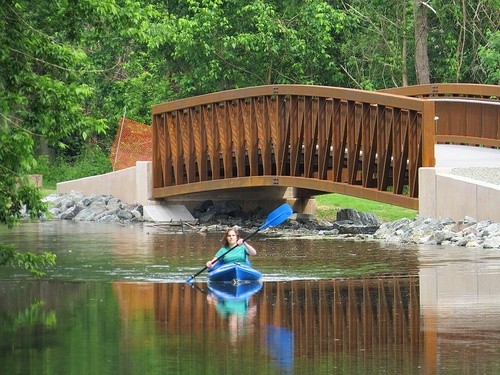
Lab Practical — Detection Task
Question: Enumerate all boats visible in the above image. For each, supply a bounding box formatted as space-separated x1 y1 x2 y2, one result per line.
207 263 262 280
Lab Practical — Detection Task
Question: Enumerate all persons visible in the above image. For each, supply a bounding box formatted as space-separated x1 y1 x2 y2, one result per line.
206 228 257 268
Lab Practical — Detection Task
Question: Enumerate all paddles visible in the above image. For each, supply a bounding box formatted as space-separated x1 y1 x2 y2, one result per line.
186 203 293 283
